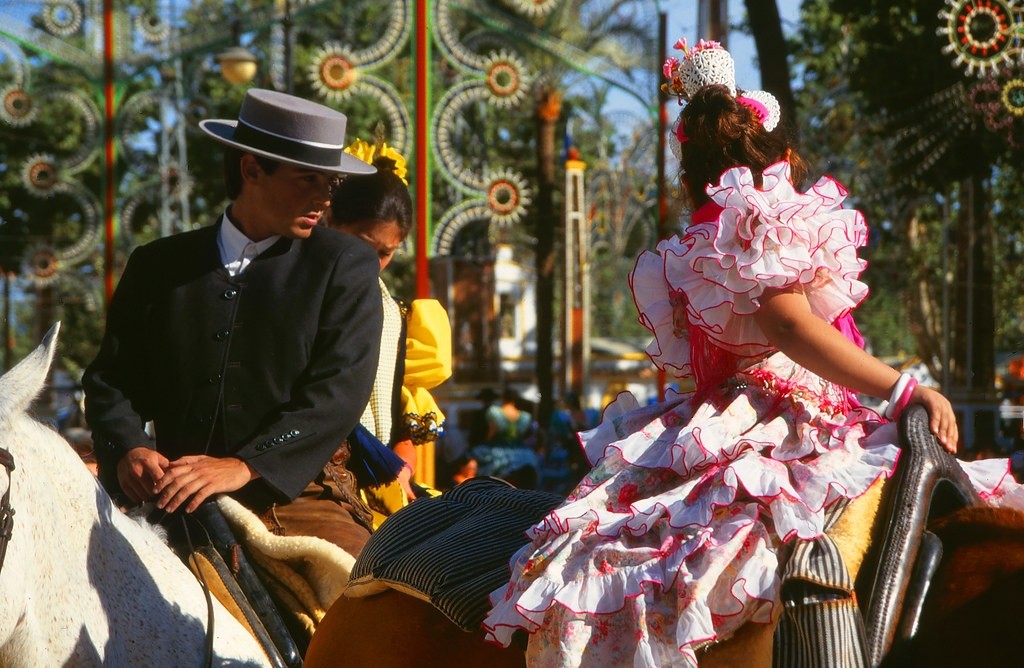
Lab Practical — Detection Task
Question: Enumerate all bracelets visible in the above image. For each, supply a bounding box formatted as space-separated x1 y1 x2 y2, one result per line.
886 373 918 423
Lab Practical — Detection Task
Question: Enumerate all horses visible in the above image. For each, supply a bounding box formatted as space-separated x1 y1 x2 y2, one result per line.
300 459 1024 668
0 322 393 667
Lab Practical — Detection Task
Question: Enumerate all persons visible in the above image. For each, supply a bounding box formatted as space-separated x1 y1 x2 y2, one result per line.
459 388 601 488
80 87 383 616
482 32 959 668
321 136 417 535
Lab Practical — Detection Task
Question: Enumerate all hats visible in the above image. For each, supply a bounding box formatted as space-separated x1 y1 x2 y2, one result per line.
662 35 782 161
198 87 378 178
